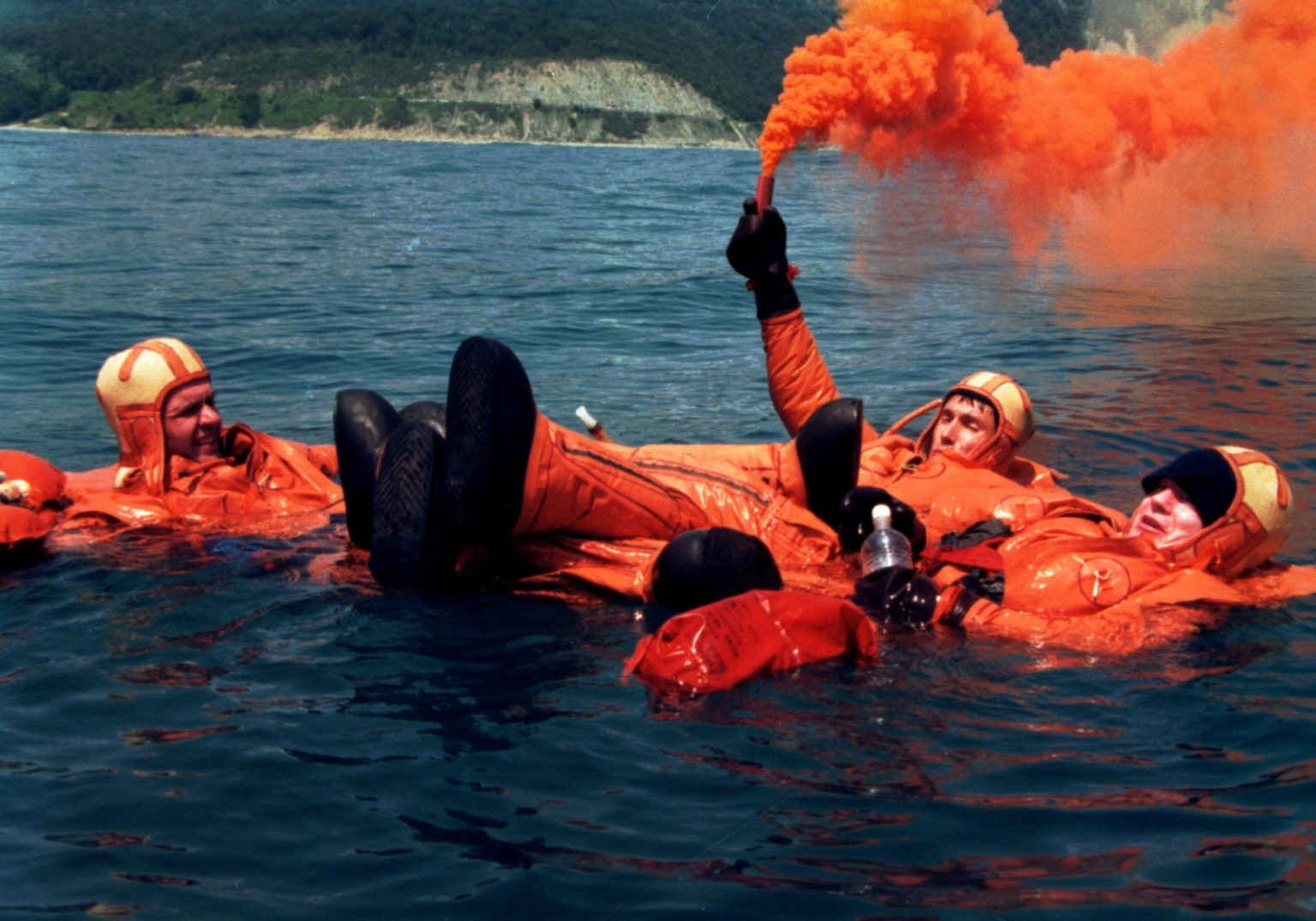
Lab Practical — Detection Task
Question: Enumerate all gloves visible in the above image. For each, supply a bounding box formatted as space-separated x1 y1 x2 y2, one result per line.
838 486 926 561
727 197 802 318
848 565 938 630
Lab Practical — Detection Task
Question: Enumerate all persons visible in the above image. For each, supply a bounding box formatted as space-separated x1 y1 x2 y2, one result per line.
46 196 1295 675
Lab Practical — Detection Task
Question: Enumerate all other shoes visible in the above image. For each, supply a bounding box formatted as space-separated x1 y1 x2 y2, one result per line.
436 338 536 550
797 398 865 535
333 389 403 545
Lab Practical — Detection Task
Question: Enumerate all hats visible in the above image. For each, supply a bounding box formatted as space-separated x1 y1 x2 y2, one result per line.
1142 448 1237 528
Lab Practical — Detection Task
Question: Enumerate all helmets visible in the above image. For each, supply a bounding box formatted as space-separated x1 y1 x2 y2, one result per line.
1167 445 1296 580
915 371 1037 474
96 336 210 497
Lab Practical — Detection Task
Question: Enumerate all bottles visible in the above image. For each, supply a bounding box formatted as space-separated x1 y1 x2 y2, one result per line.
859 502 914 576
